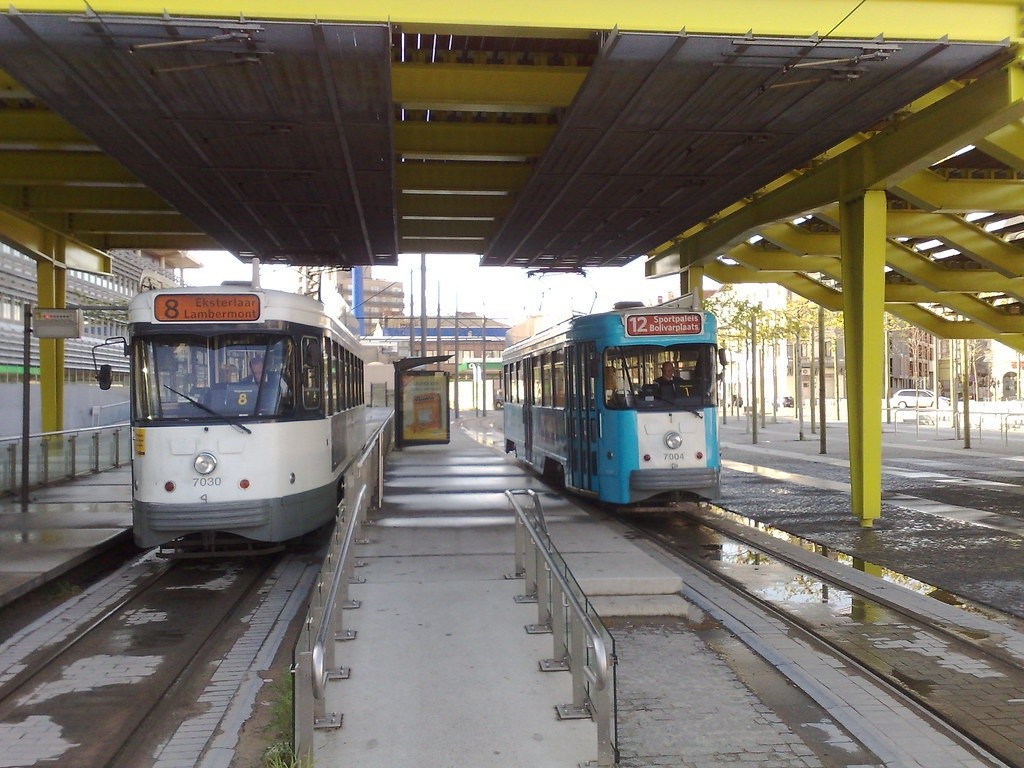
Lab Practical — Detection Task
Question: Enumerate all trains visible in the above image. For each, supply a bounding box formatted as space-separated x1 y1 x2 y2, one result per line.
125 285 369 561
501 300 728 510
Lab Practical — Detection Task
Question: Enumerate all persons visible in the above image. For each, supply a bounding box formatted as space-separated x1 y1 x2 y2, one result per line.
243 357 288 399
653 363 687 396
938 380 945 398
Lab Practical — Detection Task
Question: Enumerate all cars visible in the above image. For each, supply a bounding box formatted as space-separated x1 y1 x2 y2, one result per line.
771 396 794 408
891 388 945 409
